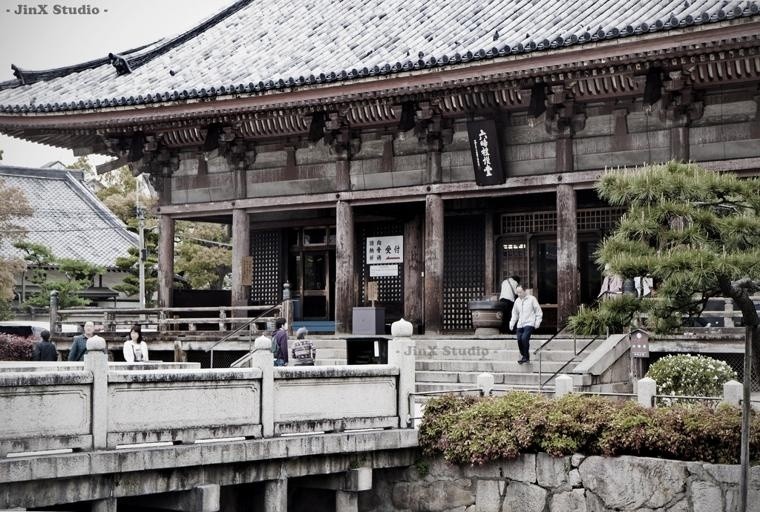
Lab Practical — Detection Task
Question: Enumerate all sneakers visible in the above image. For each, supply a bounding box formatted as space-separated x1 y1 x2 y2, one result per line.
518 358 529 362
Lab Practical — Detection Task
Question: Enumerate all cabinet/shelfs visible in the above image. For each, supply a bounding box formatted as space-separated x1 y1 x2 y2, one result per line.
352 306 385 335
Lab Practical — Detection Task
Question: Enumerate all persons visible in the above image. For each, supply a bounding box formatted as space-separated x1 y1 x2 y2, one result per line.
290 327 317 367
499 275 519 335
68 321 96 361
596 262 713 338
122 325 149 363
31 329 58 363
509 284 544 365
269 318 289 367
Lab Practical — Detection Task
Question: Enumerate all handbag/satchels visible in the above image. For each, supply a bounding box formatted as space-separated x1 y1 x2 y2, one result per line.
271 337 280 353
